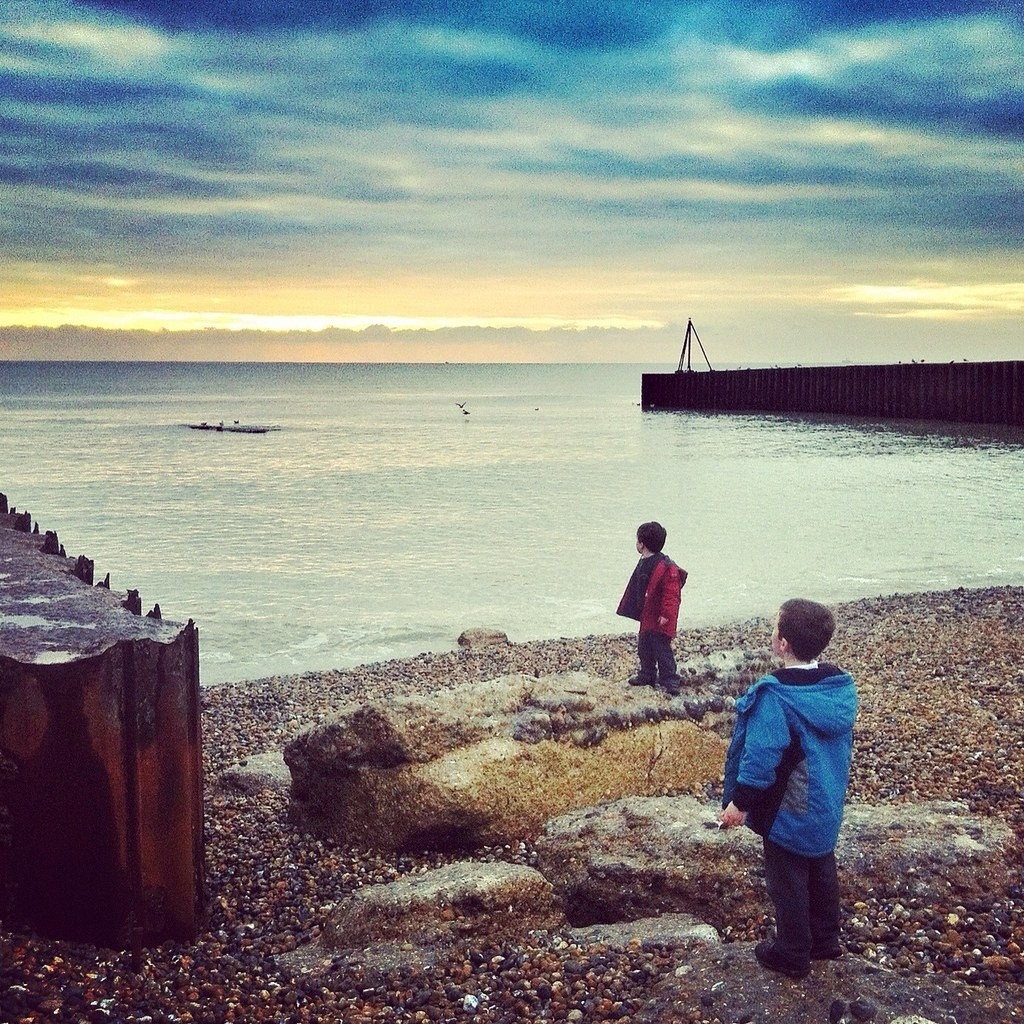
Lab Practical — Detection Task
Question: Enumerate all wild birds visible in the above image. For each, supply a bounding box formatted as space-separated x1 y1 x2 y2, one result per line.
455 402 467 408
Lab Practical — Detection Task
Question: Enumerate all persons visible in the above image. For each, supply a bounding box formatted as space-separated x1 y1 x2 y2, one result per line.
719 598 859 977
614 521 682 694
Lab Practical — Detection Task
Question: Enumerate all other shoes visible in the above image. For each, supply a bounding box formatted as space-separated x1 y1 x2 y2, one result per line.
810 945 843 959
755 942 811 978
665 682 680 696
628 675 656 688
